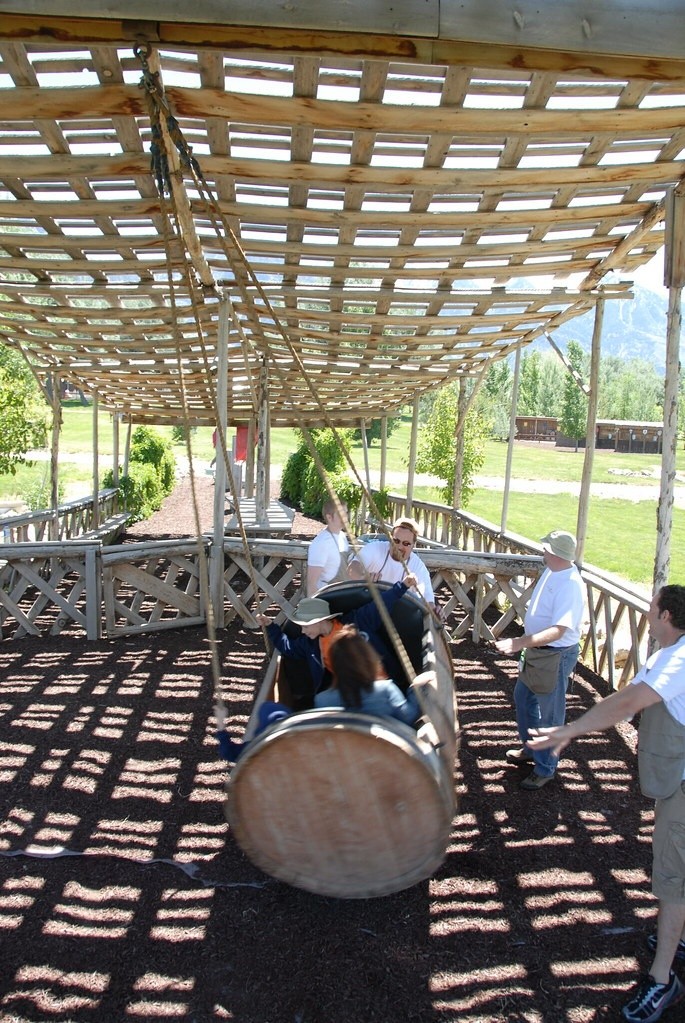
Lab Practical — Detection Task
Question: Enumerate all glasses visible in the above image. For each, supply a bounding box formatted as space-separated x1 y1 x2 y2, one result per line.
391 537 413 546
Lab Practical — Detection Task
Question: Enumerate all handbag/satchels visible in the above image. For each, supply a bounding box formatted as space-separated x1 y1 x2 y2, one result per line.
515 646 561 694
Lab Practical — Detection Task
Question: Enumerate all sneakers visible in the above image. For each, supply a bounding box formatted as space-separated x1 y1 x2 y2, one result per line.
505 748 534 762
618 968 684 1023
647 934 685 958
520 771 554 790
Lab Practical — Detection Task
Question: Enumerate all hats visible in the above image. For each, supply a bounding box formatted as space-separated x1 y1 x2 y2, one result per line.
392 518 420 538
283 598 343 626
540 529 577 561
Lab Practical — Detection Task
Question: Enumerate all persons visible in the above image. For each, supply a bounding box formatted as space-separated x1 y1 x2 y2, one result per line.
346 522 447 621
307 500 350 598
526 586 685 1023
495 530 586 789
255 573 434 724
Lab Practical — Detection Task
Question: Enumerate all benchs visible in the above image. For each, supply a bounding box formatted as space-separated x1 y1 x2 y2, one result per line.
67 512 132 546
